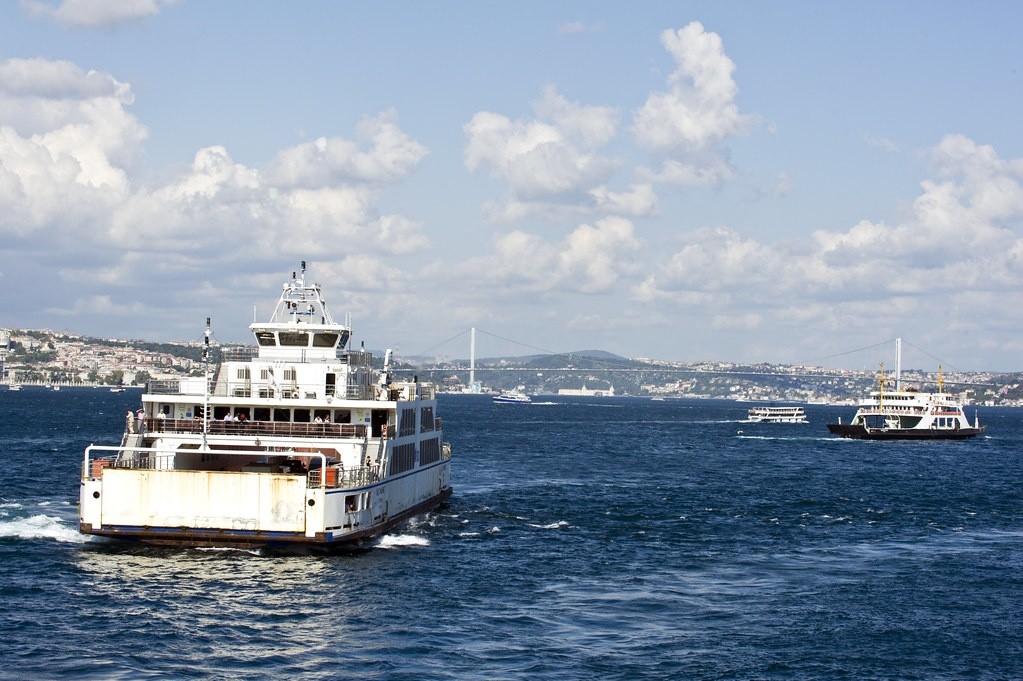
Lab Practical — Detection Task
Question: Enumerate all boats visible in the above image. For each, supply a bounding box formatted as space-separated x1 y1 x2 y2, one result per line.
77 258 455 546
747 407 811 424
826 361 988 440
492 386 533 405
